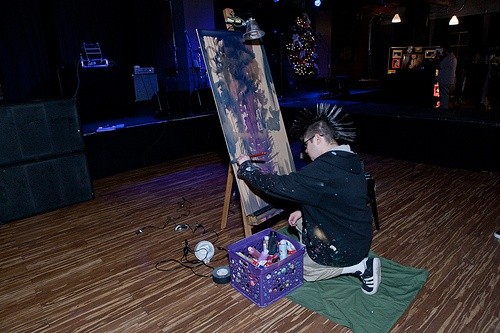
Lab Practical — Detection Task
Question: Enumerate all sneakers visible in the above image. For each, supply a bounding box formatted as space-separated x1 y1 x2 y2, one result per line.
359 257 381 295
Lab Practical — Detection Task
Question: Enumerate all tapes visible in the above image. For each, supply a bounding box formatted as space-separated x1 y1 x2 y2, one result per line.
213 265 230 284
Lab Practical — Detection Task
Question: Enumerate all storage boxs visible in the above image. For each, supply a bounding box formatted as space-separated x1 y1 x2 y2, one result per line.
228 228 304 307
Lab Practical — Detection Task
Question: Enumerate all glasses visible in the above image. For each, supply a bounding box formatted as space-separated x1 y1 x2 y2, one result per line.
303 134 324 148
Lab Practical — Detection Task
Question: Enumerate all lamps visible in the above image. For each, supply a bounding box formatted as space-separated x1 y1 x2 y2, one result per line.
227 10 264 40
391 14 402 23
448 0 459 26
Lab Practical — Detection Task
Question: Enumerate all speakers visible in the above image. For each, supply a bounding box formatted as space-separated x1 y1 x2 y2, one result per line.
0 99 95 225
263 40 289 97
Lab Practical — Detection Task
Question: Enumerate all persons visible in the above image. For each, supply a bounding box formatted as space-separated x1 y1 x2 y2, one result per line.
436 44 457 111
236 102 381 295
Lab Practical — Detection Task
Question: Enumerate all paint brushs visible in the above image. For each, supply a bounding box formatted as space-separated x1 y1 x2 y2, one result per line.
229 152 267 165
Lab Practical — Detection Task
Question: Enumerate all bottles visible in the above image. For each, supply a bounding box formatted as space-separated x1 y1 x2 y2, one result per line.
268 231 278 263
279 240 288 261
263 235 270 252
247 246 260 260
